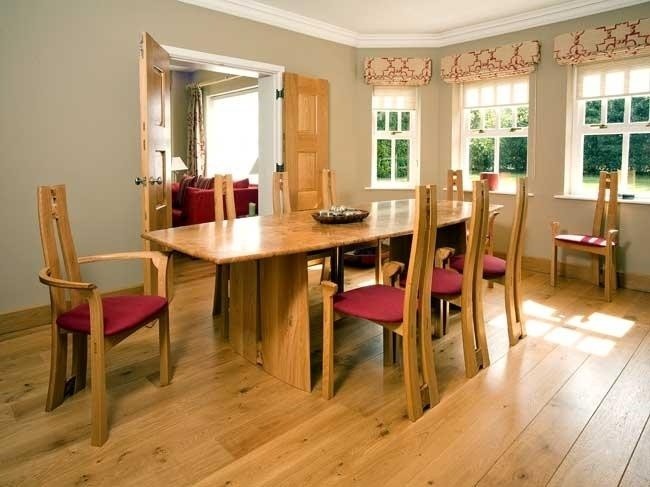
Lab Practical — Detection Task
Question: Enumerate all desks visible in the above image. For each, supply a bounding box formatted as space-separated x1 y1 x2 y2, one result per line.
142 199 503 392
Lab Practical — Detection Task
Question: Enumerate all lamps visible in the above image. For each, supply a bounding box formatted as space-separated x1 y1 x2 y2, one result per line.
250 158 258 174
172 156 188 182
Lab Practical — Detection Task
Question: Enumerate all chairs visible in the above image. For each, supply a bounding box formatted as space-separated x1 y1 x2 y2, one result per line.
321 186 439 421
318 169 337 208
39 183 173 446
392 178 490 377
444 169 465 201
271 171 292 214
446 174 531 346
550 171 619 302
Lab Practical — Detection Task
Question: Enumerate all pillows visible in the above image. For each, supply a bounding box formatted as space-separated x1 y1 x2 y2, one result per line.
177 174 248 208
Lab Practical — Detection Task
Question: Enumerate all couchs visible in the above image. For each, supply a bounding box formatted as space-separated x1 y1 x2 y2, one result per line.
172 183 258 225
